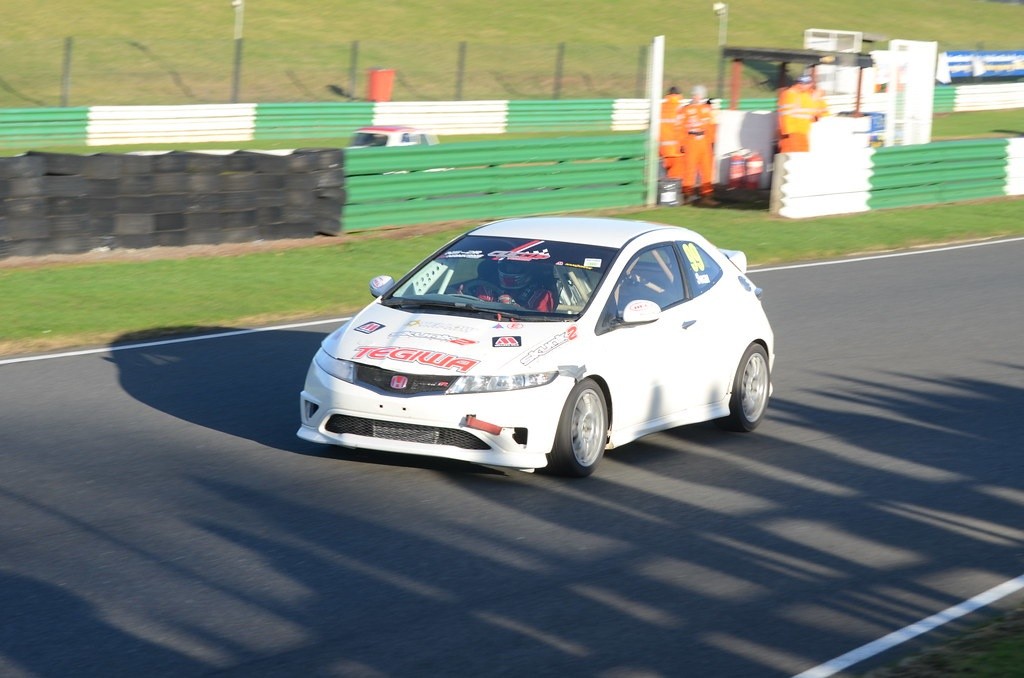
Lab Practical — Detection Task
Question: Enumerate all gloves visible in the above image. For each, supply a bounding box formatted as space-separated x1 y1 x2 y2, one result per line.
498 294 519 308
457 284 465 295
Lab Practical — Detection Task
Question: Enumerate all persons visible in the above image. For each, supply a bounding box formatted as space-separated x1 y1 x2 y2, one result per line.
776 74 827 152
457 259 554 312
659 85 721 206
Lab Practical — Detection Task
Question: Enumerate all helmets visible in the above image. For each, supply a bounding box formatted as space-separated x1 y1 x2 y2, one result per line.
498 259 530 290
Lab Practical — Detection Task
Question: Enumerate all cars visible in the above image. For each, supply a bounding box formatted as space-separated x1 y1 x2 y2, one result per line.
296 216 776 479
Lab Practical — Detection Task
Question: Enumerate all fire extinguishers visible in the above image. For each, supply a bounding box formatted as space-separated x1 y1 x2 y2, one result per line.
724 148 763 190
